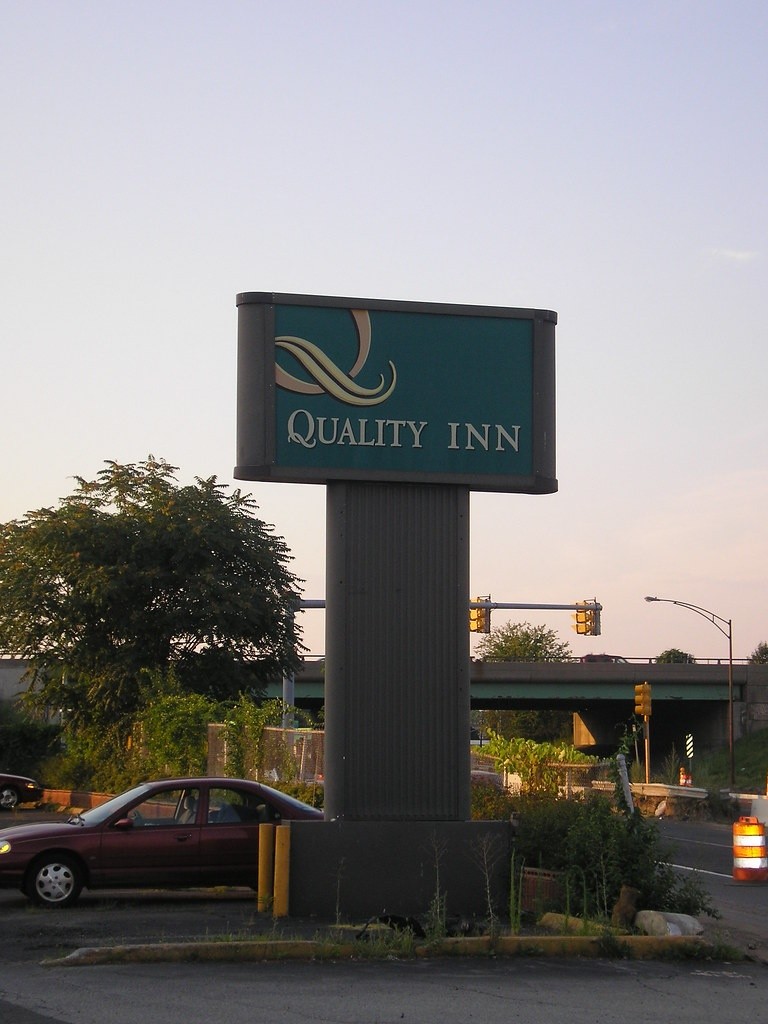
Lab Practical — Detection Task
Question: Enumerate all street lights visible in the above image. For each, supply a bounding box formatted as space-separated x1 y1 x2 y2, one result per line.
644 596 733 792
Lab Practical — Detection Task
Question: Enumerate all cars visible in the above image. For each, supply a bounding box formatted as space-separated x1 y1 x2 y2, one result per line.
0 774 43 811
0 776 324 910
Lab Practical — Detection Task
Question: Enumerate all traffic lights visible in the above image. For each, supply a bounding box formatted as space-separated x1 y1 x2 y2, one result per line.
571 602 595 636
635 684 651 716
469 599 486 633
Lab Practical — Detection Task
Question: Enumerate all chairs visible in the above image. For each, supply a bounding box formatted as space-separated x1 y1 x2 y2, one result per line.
177 795 195 824
186 798 199 824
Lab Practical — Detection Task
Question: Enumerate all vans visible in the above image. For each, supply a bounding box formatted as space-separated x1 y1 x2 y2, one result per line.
582 654 629 663
